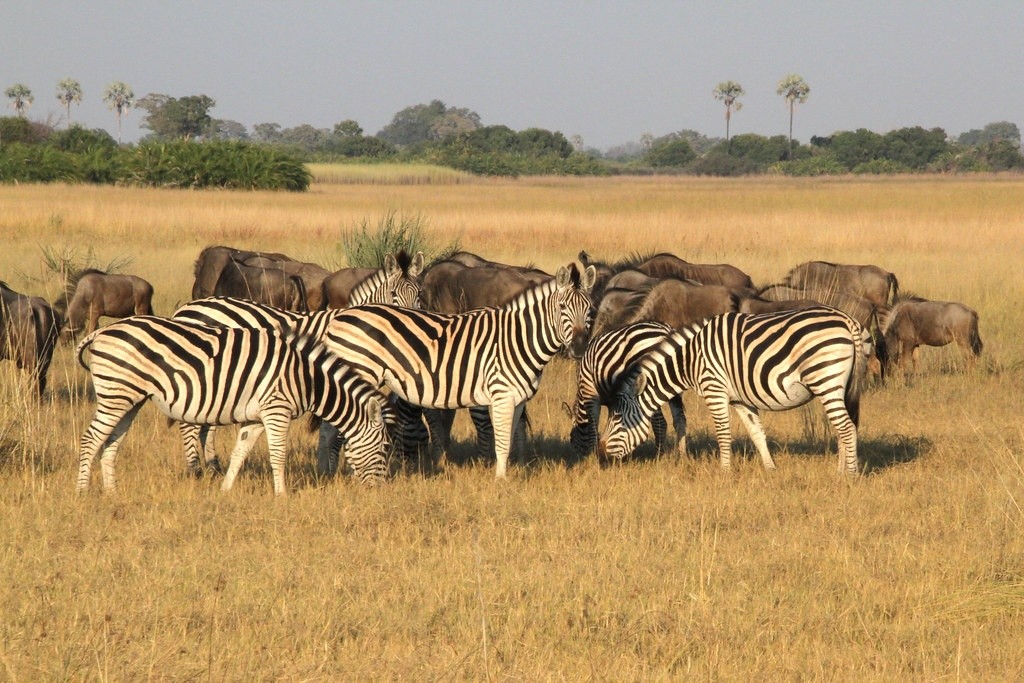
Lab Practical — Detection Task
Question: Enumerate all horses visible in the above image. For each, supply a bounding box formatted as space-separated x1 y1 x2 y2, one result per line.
1 244 983 399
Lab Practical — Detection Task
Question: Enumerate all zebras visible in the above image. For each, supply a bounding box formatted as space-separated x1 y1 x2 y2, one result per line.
73 250 867 493
